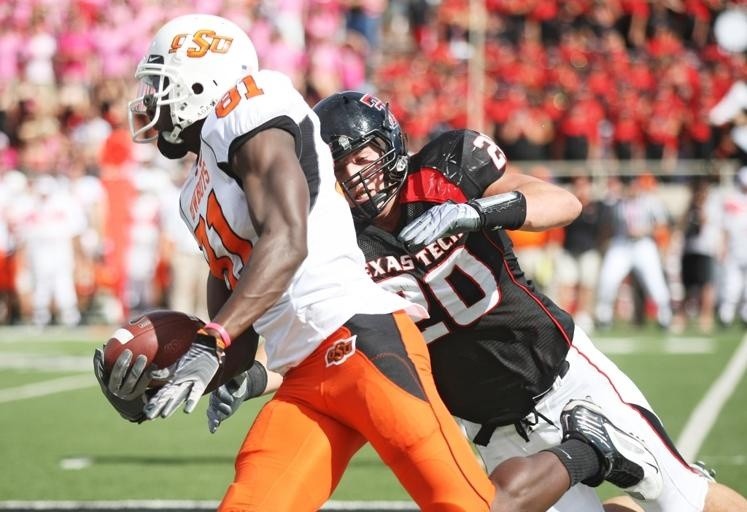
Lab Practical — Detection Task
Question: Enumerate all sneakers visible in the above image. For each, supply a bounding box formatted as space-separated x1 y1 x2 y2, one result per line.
559 399 664 503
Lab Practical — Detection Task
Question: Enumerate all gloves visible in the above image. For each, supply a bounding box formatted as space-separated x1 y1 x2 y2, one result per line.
398 202 483 247
142 342 221 419
93 347 155 423
207 358 269 434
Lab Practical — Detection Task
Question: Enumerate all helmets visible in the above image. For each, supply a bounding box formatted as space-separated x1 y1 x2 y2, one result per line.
312 91 407 226
127 12 259 160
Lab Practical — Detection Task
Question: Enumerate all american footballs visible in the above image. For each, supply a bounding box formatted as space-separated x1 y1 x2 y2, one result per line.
103 310 206 388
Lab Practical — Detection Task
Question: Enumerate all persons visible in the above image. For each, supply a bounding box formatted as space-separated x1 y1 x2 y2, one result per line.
206 90 747 512
1 3 747 331
95 14 664 512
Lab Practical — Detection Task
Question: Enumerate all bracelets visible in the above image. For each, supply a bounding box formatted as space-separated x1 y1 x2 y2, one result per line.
204 322 233 347
